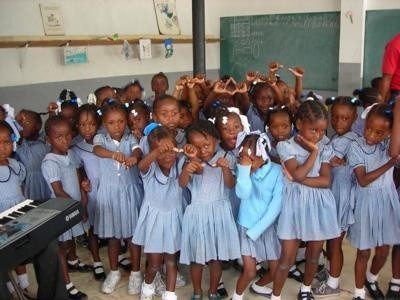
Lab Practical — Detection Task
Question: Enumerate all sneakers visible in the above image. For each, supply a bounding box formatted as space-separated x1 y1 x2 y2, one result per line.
174 271 186 289
162 289 178 300
101 270 121 294
128 270 144 295
312 281 340 297
141 280 155 300
153 271 166 296
18 282 37 299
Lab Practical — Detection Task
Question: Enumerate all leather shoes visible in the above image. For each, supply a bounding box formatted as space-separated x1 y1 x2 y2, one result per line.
364 276 385 299
92 265 107 281
192 289 203 300
117 258 132 269
254 263 267 278
67 257 94 272
297 288 315 300
232 259 244 274
217 283 228 297
65 284 87 300
249 284 273 298
288 266 305 283
352 293 365 300
386 280 400 300
208 287 221 300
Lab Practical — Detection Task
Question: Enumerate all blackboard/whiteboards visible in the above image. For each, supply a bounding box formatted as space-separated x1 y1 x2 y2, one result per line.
363 9 400 87
220 11 340 91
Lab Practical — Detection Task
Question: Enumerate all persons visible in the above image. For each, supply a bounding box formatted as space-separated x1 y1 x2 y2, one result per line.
144 73 169 113
347 103 399 300
215 107 251 271
375 39 400 103
13 109 52 199
88 85 120 125
128 102 151 141
174 61 304 116
0 120 38 300
311 96 358 297
112 88 130 111
389 94 400 300
271 94 342 300
92 103 142 294
41 116 95 300
139 94 192 294
176 100 192 129
0 106 23 154
265 105 294 166
246 84 275 133
60 100 84 148
370 77 384 93
352 89 384 112
137 126 203 300
71 104 133 280
125 79 145 102
178 118 241 300
229 130 283 300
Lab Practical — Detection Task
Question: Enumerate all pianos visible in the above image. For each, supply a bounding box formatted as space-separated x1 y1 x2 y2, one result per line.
0 196 86 271
